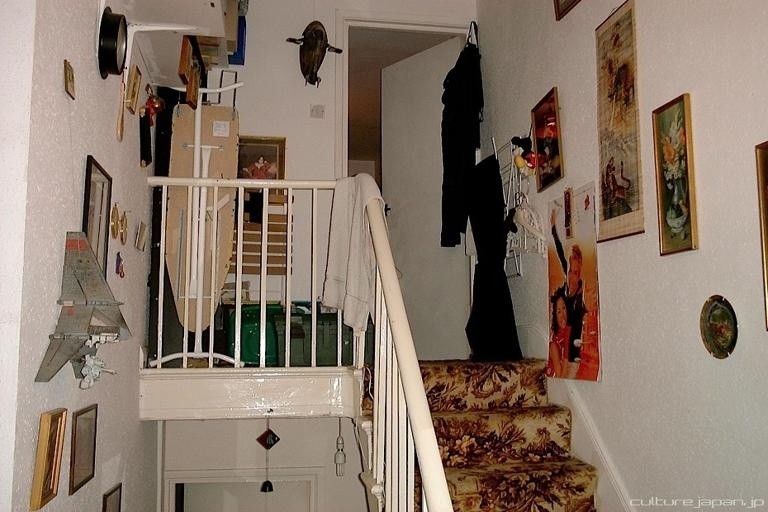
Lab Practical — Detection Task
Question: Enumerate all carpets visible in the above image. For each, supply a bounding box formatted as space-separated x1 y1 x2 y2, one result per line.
361 358 601 512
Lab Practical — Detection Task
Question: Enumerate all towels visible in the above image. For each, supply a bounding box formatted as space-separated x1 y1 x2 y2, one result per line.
320 175 389 337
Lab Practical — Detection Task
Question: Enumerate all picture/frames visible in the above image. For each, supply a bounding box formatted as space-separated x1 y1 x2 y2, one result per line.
31 402 122 511
63 58 75 102
124 64 142 115
530 87 565 193
238 135 286 206
651 92 700 257
82 154 112 282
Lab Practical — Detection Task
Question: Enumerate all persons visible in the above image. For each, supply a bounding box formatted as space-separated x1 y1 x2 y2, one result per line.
549 291 573 379
243 154 278 224
548 206 587 380
539 121 555 175
